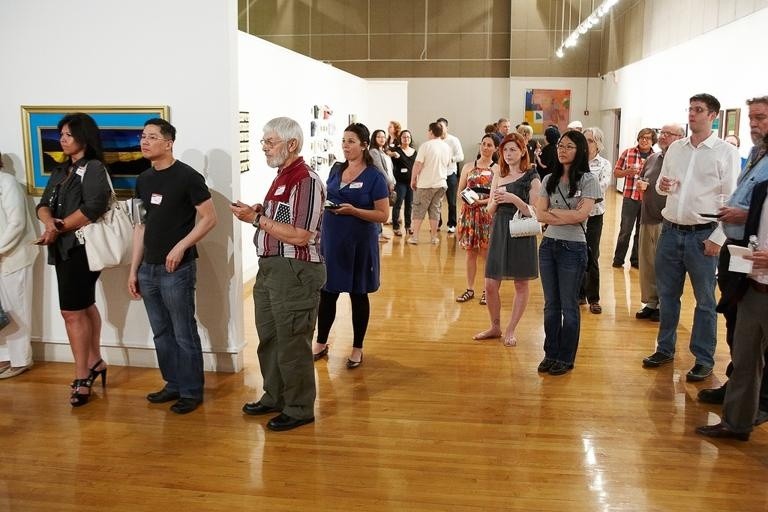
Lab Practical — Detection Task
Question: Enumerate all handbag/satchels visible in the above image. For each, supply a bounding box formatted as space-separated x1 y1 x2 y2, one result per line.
585 232 599 271
509 216 542 239
75 197 133 273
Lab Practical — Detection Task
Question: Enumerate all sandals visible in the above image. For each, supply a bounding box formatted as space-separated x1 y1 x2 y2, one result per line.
456 288 474 302
479 290 486 305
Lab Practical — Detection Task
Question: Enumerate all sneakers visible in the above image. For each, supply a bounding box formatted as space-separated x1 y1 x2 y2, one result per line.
472 330 502 340
643 352 674 367
578 299 587 304
590 302 601 313
613 262 622 267
687 363 713 381
538 358 573 375
636 307 660 322
632 263 639 269
503 336 516 347
375 219 456 244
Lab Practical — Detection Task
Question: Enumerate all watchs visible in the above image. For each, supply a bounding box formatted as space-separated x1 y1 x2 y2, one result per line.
251 213 262 228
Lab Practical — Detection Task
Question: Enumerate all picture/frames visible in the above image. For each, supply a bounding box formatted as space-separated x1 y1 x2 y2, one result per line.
712 110 723 138
725 108 741 137
523 88 572 136
20 105 169 201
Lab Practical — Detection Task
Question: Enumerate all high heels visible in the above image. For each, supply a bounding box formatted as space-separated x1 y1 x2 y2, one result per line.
70 359 106 406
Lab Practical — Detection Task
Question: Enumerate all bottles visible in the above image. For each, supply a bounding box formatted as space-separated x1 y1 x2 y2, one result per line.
748 234 763 252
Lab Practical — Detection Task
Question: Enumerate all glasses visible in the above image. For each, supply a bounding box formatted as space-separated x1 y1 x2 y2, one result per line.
640 135 653 141
660 131 681 138
555 143 577 150
137 133 169 143
260 139 289 149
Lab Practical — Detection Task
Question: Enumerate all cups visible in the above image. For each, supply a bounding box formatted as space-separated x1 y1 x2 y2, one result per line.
497 186 506 191
667 176 679 192
714 194 729 216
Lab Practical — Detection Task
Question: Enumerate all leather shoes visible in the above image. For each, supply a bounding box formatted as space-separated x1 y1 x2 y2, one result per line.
754 409 768 425
266 413 315 430
0 359 33 378
170 398 203 414
243 401 278 415
696 421 749 441
313 345 328 361
697 380 728 404
346 349 363 369
146 387 181 403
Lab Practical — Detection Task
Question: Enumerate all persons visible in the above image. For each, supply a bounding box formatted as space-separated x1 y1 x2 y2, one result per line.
478 120 585 176
129 119 217 413
473 133 541 348
229 117 326 431
613 94 745 382
31 112 114 408
370 117 465 246
696 94 768 440
455 134 501 306
576 127 613 314
0 150 38 379
537 130 603 374
314 122 389 368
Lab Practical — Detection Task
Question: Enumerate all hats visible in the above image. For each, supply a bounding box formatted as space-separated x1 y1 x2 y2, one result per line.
567 120 582 129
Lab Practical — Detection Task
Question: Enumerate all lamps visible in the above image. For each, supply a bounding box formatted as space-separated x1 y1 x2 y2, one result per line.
555 0 619 58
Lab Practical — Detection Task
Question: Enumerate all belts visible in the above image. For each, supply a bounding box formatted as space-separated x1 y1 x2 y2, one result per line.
747 278 768 294
665 219 717 232
